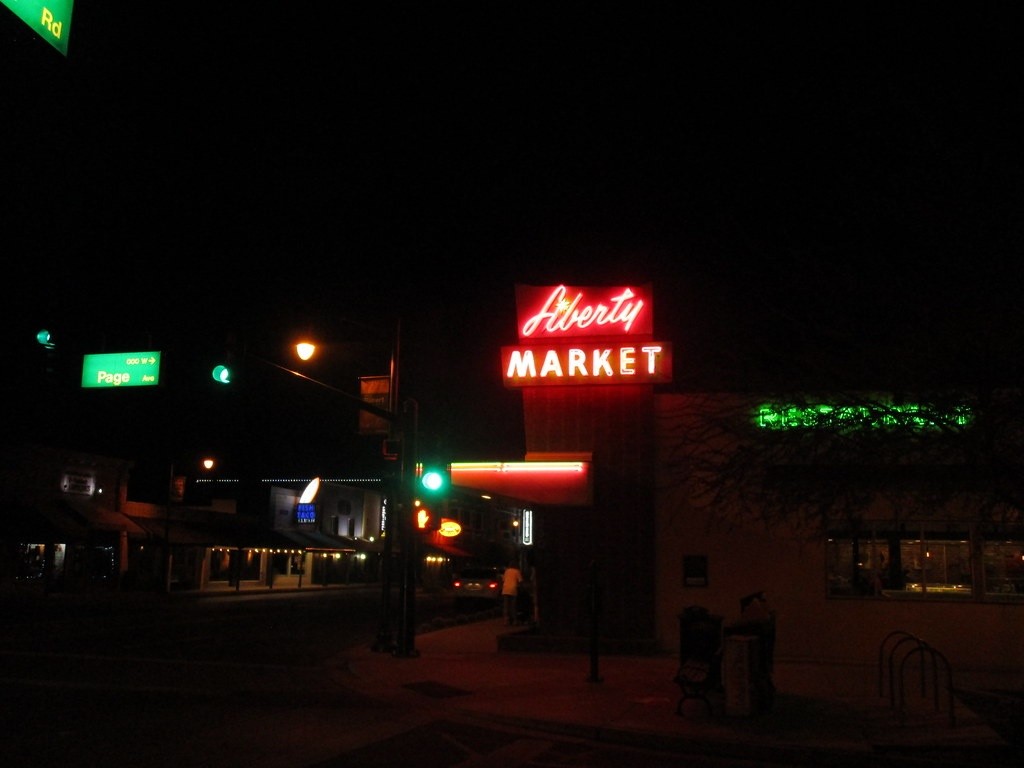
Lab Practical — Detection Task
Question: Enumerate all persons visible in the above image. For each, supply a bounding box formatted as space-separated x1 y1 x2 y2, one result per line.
498 559 532 626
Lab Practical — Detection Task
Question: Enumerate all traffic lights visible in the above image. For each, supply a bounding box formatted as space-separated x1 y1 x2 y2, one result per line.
209 362 233 386
418 467 449 498
35 327 55 349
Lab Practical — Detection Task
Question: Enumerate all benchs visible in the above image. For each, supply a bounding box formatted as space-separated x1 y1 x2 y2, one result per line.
673 644 727 718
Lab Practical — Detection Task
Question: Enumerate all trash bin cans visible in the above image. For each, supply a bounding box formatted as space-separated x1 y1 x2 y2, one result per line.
678 606 720 693
720 613 776 719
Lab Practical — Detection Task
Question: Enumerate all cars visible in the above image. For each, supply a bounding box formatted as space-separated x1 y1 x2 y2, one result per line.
449 567 502 609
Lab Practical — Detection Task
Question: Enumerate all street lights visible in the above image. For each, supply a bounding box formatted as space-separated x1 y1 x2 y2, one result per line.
165 457 215 592
294 338 399 651
